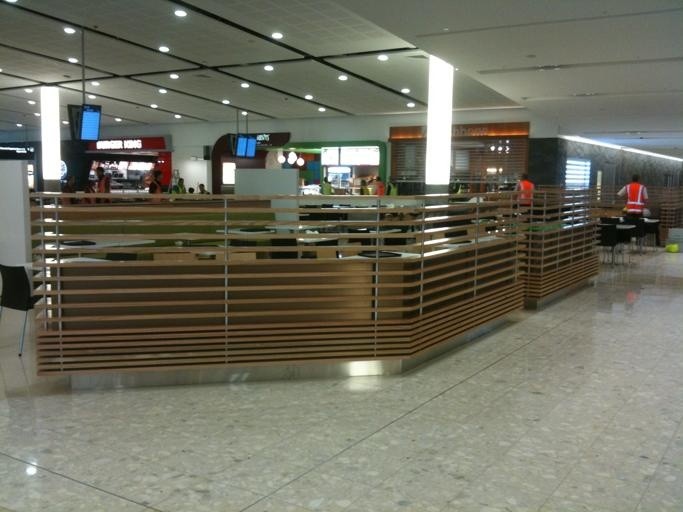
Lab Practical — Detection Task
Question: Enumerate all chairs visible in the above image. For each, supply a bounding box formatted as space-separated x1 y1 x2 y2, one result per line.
0 264 43 356
595 207 661 266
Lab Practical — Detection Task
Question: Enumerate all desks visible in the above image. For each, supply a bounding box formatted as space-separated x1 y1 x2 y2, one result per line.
341 234 517 356
216 225 349 259
31 239 155 257
518 217 593 298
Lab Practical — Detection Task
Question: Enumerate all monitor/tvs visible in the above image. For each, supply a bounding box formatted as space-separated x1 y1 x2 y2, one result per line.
78 103 101 141
67 104 82 140
227 133 257 158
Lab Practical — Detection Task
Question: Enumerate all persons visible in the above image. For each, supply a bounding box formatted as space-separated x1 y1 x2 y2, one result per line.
148 170 211 194
92 168 109 194
320 177 332 194
616 174 649 223
516 173 536 215
360 176 398 196
